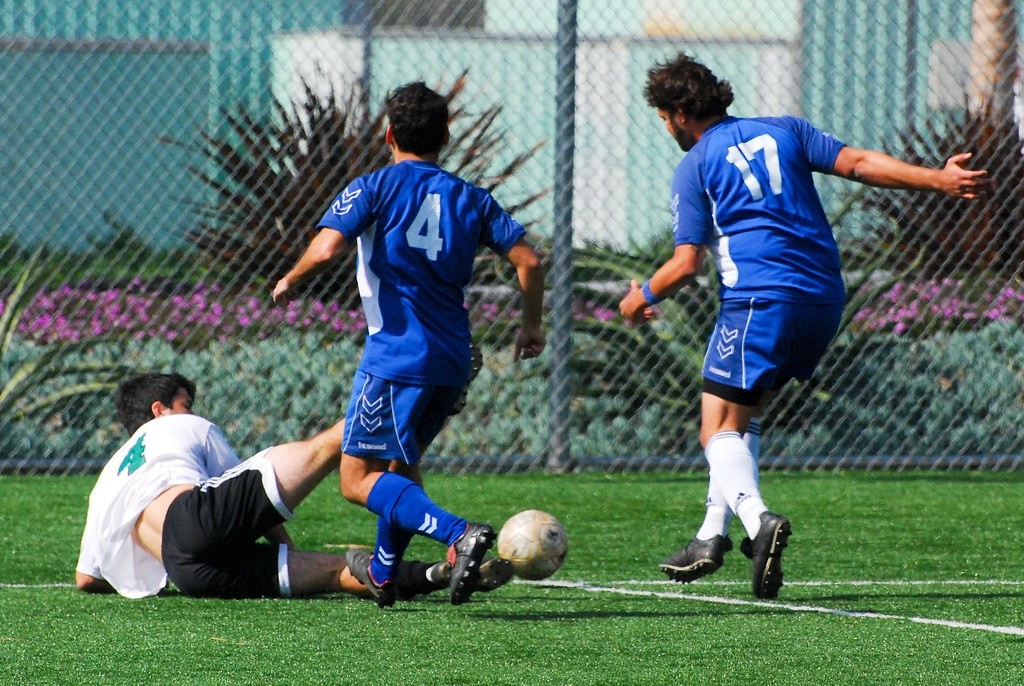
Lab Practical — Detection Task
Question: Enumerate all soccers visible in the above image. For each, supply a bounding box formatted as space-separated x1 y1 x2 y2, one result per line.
496 507 570 581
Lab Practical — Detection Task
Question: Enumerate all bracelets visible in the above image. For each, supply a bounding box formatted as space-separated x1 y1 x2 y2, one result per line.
642 280 658 305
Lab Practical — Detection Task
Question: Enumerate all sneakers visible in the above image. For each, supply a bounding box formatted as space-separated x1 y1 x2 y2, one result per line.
446 343 483 417
658 534 733 585
740 511 792 599
346 550 396 609
431 557 514 592
446 522 498 605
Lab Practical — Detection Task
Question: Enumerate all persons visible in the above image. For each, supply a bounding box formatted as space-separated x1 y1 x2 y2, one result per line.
72 372 514 599
269 80 546 611
618 49 995 600
